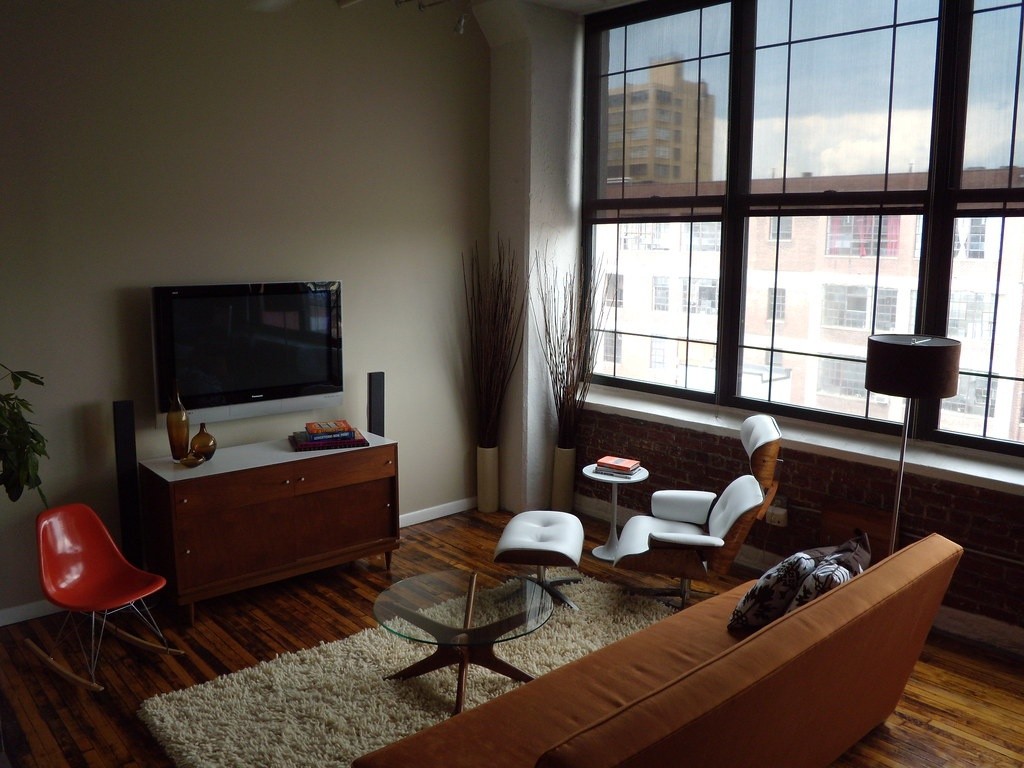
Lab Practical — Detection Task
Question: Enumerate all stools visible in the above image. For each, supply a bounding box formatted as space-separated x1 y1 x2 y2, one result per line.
493 511 585 611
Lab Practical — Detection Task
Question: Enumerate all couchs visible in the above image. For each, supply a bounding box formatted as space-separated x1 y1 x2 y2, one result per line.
352 532 963 768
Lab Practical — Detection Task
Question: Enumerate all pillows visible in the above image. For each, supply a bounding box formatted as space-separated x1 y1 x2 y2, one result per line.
727 546 838 632
784 533 872 613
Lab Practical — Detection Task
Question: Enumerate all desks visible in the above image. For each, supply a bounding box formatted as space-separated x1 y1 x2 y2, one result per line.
582 463 649 561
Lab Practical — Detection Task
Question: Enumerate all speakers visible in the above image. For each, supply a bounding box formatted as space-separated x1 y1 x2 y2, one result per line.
112 399 157 612
367 371 385 439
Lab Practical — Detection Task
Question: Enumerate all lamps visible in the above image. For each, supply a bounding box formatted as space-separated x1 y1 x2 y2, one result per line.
865 334 960 555
418 0 471 34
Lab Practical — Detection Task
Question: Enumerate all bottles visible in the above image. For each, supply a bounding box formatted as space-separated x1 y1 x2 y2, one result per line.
180 448 205 468
167 379 190 464
191 423 216 460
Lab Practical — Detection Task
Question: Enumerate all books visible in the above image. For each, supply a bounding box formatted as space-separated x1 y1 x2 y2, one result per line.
593 456 642 479
288 419 371 452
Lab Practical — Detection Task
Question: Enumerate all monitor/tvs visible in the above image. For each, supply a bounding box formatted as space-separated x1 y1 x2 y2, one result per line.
150 279 344 429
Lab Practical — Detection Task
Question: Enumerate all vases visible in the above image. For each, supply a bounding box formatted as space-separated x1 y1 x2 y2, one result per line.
476 446 499 512
551 444 575 510
181 449 205 467
190 423 217 461
167 379 189 460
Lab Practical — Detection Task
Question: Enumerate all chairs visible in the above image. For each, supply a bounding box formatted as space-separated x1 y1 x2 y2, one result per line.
614 415 783 610
23 503 185 692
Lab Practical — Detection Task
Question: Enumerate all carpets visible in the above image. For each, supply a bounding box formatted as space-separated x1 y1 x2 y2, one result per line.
135 564 678 768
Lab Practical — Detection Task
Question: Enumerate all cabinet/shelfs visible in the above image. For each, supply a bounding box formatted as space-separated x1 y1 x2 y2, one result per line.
139 428 400 628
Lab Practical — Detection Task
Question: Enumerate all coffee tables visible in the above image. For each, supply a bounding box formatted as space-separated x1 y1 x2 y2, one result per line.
374 569 554 716
